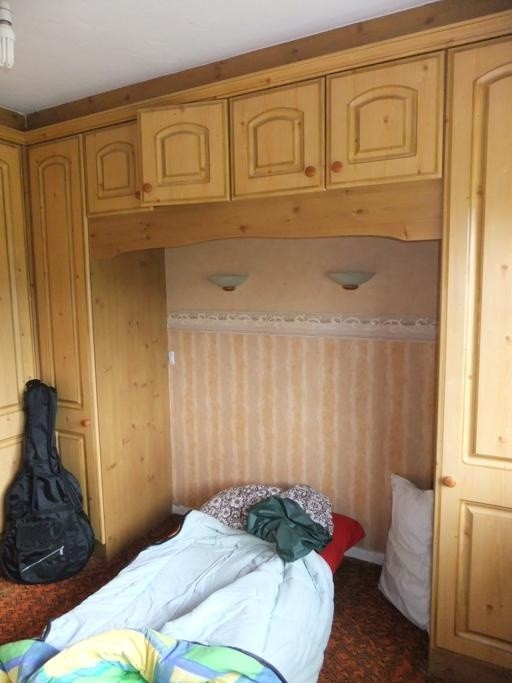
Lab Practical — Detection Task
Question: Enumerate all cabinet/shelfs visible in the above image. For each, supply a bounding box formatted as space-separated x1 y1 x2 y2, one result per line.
138 95 228 210
323 50 441 191
0 122 39 494
80 117 153 216
228 79 324 200
23 131 107 555
428 30 512 683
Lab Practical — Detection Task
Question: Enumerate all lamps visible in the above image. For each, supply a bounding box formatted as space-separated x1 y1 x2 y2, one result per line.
0 1 14 68
326 270 375 289
206 273 248 291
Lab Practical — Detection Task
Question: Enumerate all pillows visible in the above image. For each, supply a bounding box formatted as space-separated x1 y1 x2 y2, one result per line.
378 472 436 632
320 513 365 574
